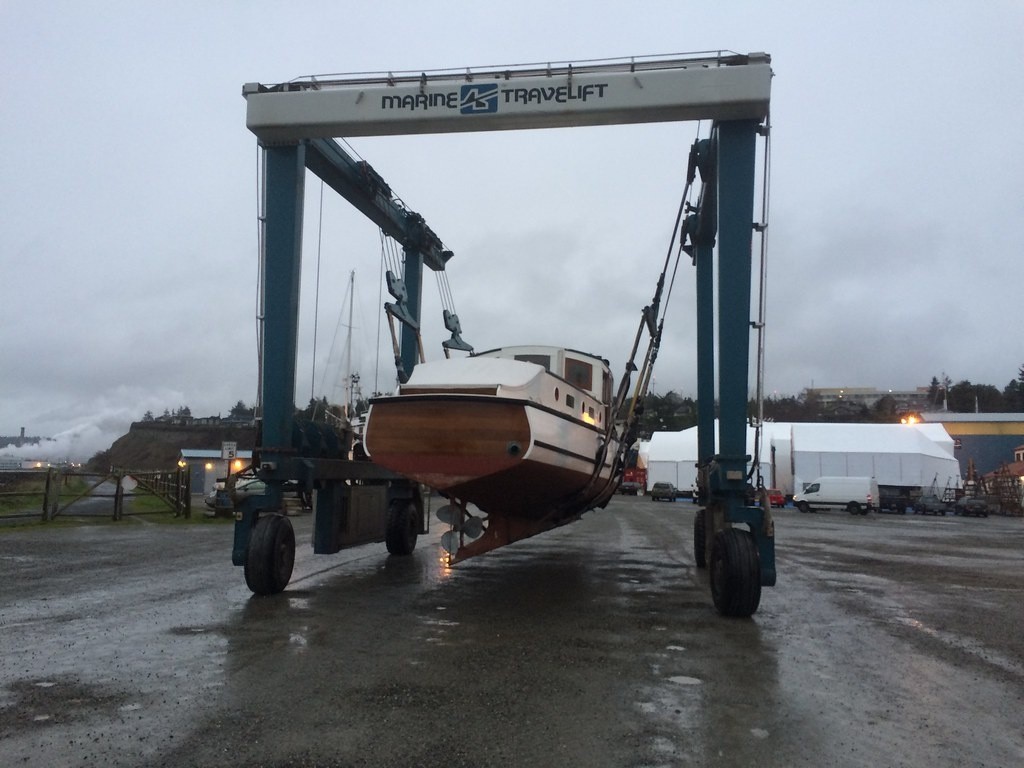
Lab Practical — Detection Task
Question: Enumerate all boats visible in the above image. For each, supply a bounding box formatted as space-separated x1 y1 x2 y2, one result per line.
360 344 623 566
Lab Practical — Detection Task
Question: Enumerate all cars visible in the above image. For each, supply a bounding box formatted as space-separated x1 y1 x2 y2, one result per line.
651 482 679 503
767 489 785 508
203 475 305 512
619 482 641 496
690 481 699 503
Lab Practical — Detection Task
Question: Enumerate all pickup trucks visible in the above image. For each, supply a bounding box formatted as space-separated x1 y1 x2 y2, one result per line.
913 496 946 517
880 487 908 513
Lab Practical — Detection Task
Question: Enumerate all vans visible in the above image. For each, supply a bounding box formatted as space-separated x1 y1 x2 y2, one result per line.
793 476 879 519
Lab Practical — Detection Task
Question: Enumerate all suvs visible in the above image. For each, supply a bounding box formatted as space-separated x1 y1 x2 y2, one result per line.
954 496 989 518
